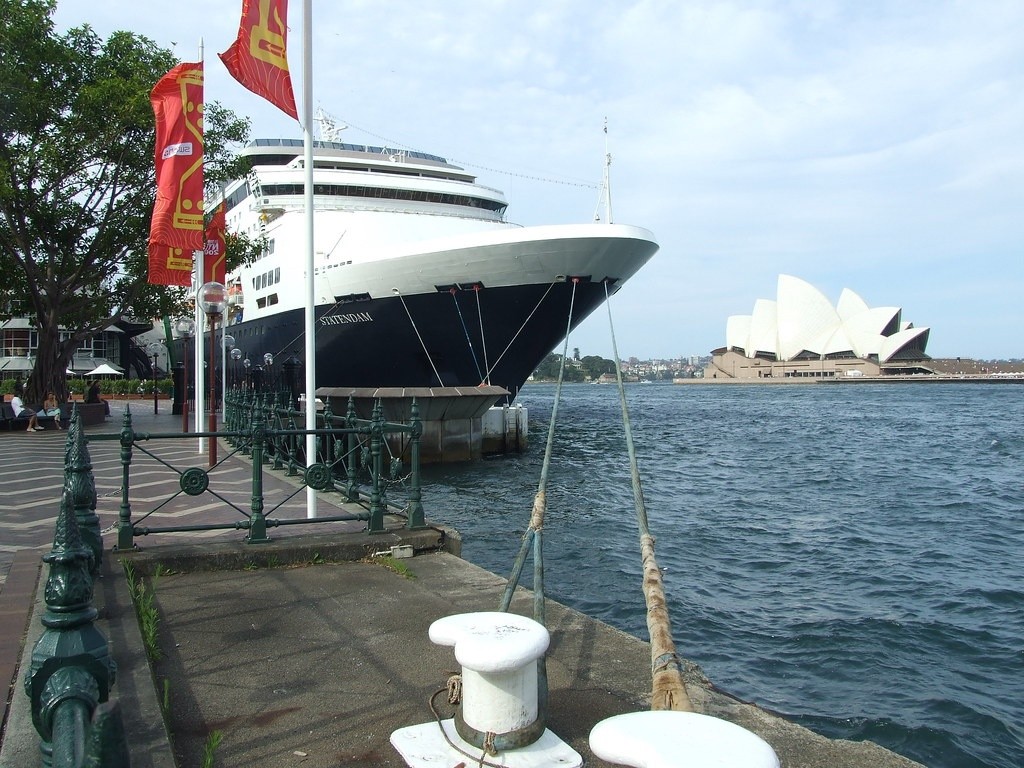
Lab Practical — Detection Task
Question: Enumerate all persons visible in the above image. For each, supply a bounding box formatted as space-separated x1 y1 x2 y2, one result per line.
14 376 30 398
137 383 146 400
44 392 62 430
152 386 161 398
12 393 45 432
84 380 113 417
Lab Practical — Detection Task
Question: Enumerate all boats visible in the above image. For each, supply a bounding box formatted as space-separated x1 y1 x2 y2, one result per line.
228 284 244 311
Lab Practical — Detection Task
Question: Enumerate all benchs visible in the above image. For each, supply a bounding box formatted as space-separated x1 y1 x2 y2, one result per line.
0 404 71 431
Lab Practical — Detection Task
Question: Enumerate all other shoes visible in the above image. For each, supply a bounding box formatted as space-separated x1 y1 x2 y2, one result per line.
34 426 44 429
26 427 36 432
59 427 61 432
106 414 113 417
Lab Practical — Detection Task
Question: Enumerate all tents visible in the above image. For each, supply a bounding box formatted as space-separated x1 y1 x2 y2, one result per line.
84 364 124 380
66 369 76 381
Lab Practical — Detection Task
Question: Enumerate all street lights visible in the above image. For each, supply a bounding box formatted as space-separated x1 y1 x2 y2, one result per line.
220 333 277 397
175 317 196 434
196 282 230 470
150 342 163 416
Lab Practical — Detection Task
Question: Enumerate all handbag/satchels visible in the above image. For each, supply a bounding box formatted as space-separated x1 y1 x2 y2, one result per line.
37 409 46 416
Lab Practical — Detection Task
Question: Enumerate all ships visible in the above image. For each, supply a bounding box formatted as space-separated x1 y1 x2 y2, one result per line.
159 103 661 474
